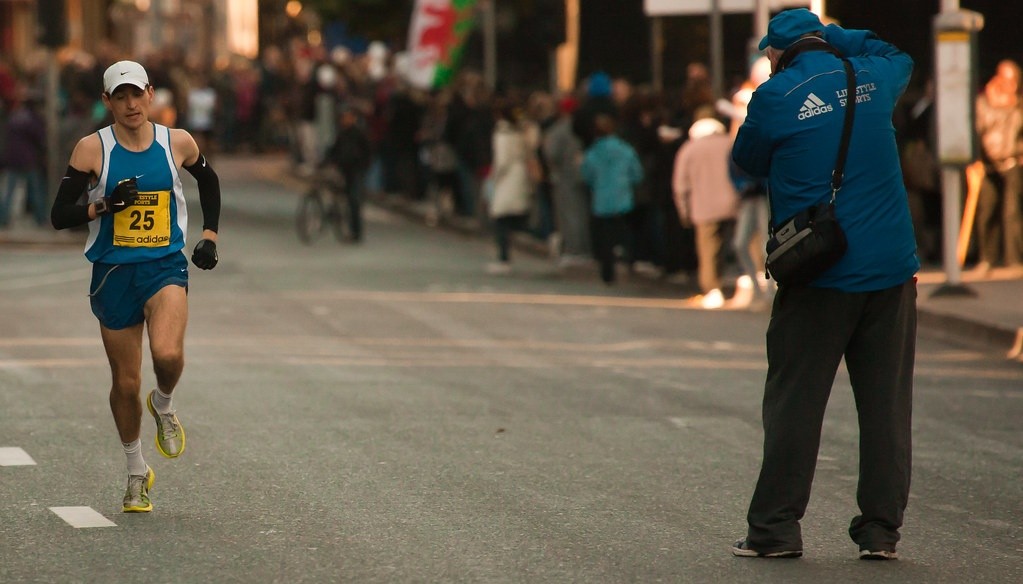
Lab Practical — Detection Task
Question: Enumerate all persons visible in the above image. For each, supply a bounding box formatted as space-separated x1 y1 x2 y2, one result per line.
728 9 919 561
0 0 1023 309
51 59 221 513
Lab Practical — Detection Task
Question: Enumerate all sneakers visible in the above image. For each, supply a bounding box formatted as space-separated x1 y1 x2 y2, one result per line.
733 536 804 559
122 464 155 512
147 389 186 459
860 543 898 562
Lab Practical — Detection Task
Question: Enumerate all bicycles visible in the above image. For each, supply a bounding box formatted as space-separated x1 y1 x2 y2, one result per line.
294 161 360 243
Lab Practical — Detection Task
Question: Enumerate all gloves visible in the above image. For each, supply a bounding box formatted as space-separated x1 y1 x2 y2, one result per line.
191 239 219 271
108 181 140 214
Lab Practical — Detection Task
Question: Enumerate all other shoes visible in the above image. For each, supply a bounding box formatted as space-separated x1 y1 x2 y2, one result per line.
487 261 514 277
549 232 564 260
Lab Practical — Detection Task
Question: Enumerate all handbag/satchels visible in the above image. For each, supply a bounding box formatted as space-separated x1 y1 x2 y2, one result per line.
764 201 849 286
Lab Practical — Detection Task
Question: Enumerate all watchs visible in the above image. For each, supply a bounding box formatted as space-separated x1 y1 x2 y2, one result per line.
94 197 110 215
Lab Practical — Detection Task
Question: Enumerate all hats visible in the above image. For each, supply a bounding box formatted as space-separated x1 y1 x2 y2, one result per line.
758 9 827 51
104 61 149 96
